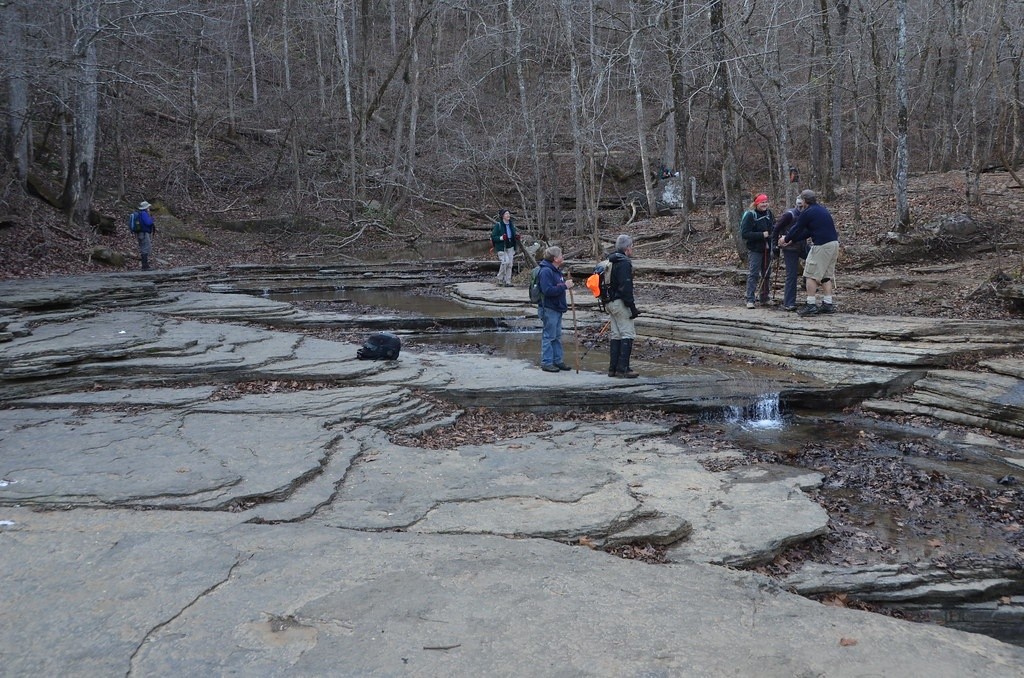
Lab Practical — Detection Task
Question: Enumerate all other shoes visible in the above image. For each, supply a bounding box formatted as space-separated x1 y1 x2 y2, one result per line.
615 370 639 378
747 302 755 308
785 305 795 311
497 279 513 287
141 264 154 271
760 300 776 306
608 370 615 377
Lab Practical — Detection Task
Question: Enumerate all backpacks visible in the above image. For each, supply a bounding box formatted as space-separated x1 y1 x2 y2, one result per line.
129 211 146 232
529 266 552 304
593 252 630 303
740 210 770 249
357 331 400 360
489 222 501 248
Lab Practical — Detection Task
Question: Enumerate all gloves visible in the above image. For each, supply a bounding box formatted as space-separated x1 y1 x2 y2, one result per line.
773 243 781 256
515 233 520 241
503 234 507 240
629 306 641 319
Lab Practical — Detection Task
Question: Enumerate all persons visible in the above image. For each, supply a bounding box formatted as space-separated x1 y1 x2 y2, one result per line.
770 195 811 311
741 193 779 309
661 160 677 179
789 160 800 183
492 208 520 287
537 246 575 372
778 189 838 316
137 201 154 271
606 235 641 378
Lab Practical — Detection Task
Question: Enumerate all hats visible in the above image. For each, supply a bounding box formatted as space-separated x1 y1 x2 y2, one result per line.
587 273 600 297
498 208 510 217
616 234 632 251
138 201 151 210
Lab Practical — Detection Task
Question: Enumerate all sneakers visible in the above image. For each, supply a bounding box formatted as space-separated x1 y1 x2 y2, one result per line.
554 362 571 370
797 302 817 317
543 364 559 372
817 301 831 313
